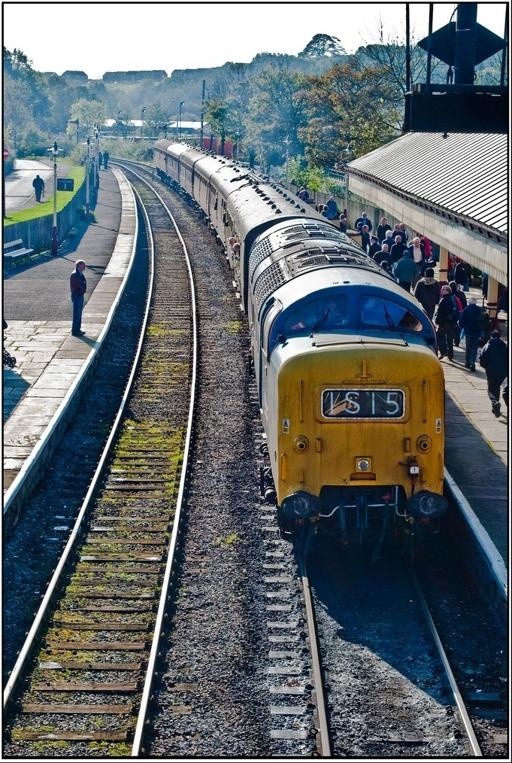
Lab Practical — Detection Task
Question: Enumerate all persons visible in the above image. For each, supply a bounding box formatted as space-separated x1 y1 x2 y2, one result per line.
71 260 87 335
103 149 110 169
296 187 439 291
33 175 44 201
414 259 508 418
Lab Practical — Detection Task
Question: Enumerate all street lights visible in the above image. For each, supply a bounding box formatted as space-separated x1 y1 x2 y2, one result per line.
141 106 147 136
117 110 123 123
178 100 185 141
83 120 102 219
47 140 65 258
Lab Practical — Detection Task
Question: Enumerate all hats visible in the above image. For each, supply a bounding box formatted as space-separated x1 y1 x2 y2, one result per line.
368 234 379 242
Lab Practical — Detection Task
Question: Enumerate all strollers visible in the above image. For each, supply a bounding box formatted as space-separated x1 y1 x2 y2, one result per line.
4 336 17 368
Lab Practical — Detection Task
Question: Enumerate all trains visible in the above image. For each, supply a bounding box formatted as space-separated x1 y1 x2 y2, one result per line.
153 135 455 564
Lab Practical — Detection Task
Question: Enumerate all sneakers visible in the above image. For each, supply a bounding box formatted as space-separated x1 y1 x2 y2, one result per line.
464 360 476 372
491 400 501 419
71 329 86 337
438 349 454 361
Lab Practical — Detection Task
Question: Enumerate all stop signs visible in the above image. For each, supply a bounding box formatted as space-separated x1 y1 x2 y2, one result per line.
4 149 8 159
